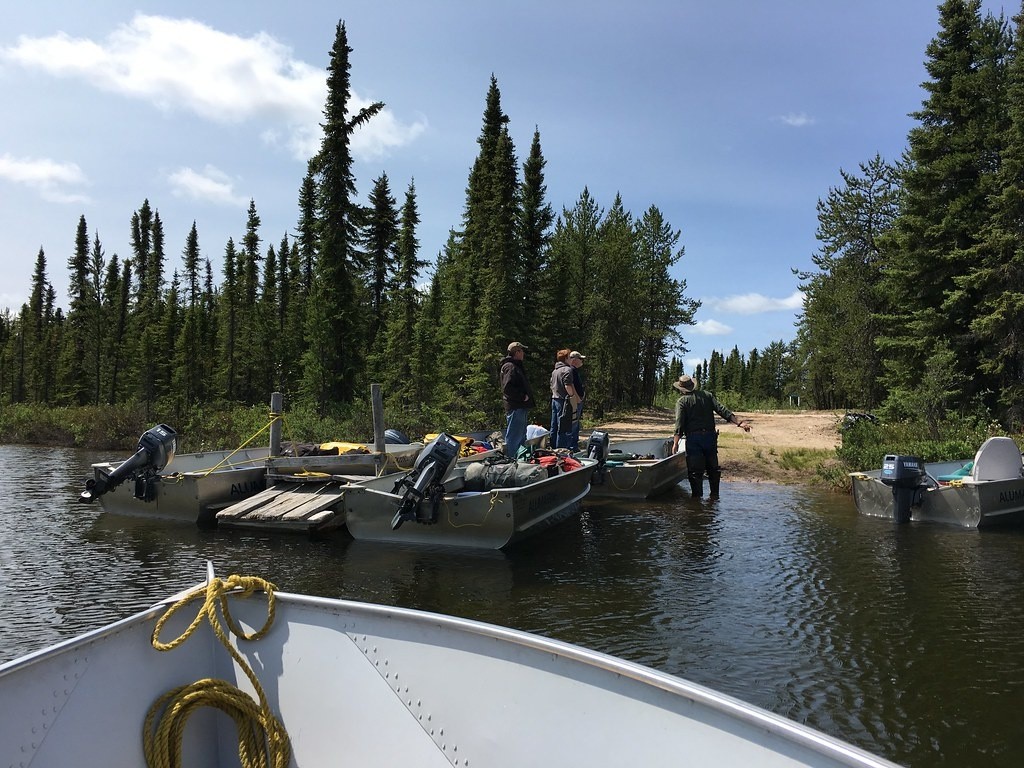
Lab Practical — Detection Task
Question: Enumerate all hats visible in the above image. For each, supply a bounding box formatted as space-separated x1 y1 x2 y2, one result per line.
570 351 586 360
673 375 698 393
506 342 529 353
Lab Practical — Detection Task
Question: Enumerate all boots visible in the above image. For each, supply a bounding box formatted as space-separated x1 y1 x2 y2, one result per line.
707 471 721 502
687 470 704 499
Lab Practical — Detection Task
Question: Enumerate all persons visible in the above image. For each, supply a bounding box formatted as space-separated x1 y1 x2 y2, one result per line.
672 375 753 501
549 349 586 453
499 342 537 458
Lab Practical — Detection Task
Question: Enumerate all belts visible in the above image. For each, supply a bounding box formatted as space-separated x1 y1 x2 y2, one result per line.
685 430 716 435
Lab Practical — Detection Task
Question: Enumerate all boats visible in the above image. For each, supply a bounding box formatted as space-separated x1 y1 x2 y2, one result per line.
339 444 600 555
428 428 550 463
850 436 1024 530
79 422 422 525
568 435 689 502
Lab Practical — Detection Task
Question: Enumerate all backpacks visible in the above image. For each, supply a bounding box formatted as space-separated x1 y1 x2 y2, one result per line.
464 448 585 491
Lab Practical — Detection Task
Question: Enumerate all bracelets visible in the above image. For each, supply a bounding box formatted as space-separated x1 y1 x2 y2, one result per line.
738 422 742 427
